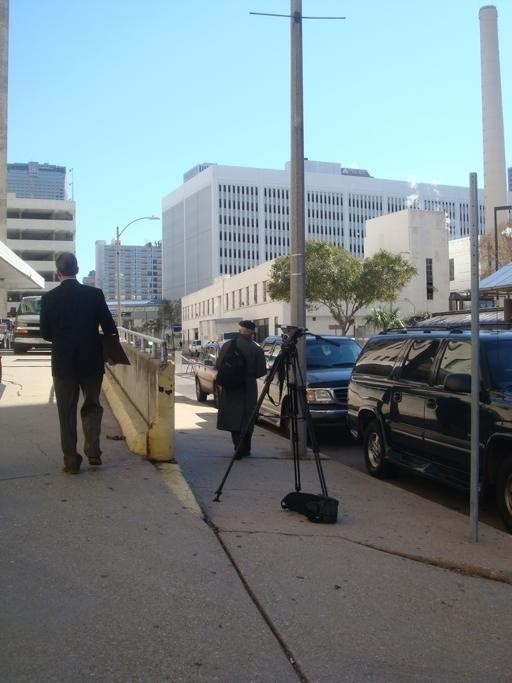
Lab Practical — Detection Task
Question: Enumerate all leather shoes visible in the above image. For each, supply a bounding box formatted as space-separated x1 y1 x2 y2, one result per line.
62 464 80 473
89 457 102 465
234 449 250 460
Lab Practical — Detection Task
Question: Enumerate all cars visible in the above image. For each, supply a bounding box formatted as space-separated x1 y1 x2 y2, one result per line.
253 336 363 438
195 337 232 401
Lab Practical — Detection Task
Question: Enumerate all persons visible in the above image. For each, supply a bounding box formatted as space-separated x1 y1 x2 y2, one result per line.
215 319 267 460
39 250 123 473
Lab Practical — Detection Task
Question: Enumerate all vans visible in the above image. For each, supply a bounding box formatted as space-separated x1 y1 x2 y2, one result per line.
0 295 52 354
347 324 512 528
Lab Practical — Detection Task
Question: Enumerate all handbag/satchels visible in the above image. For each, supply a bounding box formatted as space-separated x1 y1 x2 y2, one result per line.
281 493 339 523
215 349 248 395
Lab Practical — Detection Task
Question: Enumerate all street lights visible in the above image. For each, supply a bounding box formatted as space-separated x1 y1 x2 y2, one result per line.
113 213 160 326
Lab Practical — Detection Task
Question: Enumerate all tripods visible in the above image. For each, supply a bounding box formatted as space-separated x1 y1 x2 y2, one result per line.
213 343 328 502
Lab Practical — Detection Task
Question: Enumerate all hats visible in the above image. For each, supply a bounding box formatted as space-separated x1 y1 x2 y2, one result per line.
240 320 255 329
